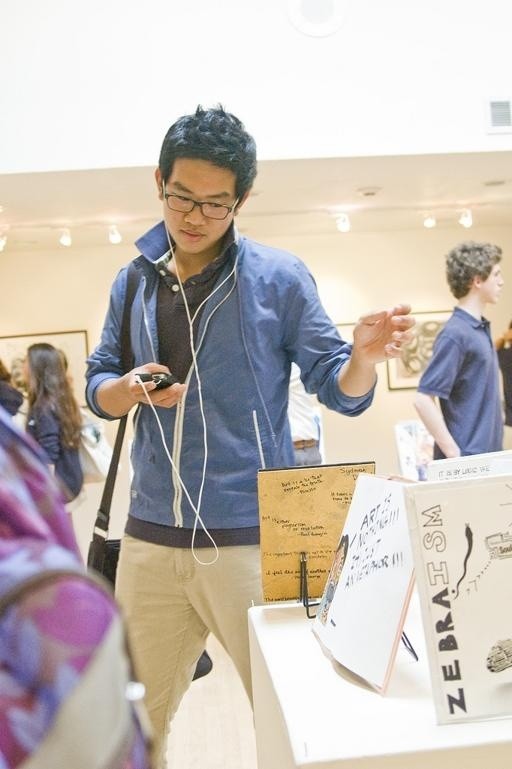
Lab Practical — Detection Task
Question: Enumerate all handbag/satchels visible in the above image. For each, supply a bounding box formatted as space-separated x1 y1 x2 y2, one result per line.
77 408 121 485
79 529 213 682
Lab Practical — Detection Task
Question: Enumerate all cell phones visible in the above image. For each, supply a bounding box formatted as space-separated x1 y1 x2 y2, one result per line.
134 372 180 390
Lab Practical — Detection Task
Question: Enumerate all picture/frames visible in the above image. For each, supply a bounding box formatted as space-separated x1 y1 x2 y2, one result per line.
386 310 458 390
0 330 91 409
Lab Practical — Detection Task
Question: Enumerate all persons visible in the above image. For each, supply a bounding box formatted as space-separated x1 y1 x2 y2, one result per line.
26 344 83 505
85 104 416 769
413 242 504 460
288 362 322 466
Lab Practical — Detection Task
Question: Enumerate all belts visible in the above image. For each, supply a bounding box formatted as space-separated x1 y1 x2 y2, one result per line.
293 439 316 450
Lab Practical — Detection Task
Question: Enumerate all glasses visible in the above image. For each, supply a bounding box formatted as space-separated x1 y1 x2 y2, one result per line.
161 178 242 220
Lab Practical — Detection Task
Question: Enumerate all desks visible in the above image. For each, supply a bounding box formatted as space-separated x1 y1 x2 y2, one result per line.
247 601 511 769
